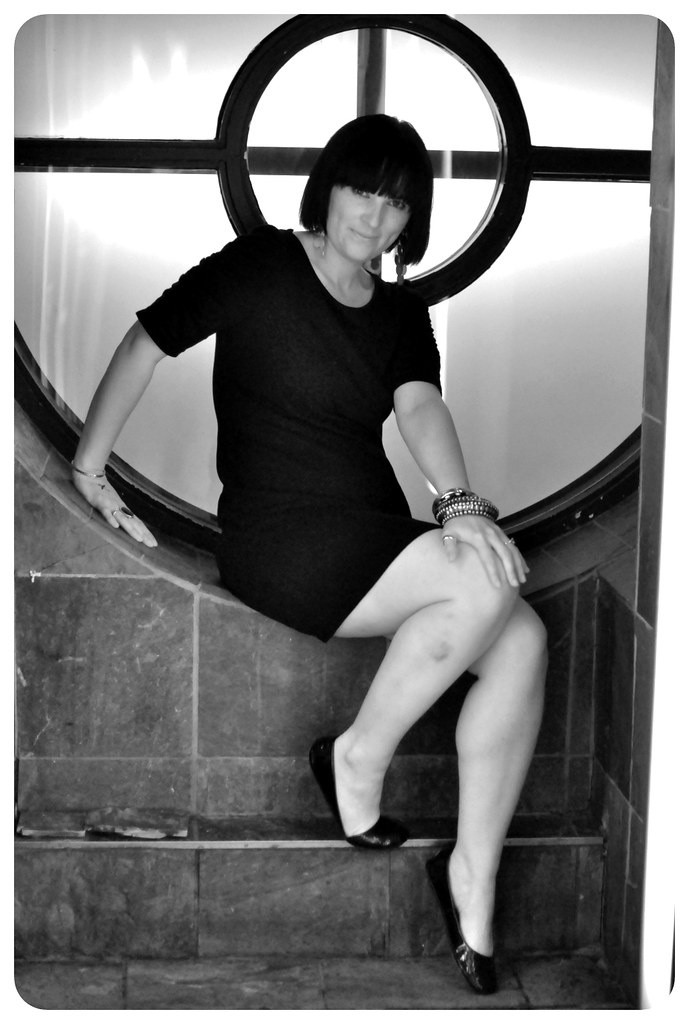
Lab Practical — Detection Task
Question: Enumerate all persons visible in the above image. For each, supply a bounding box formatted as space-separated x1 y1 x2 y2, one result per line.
69 114 548 993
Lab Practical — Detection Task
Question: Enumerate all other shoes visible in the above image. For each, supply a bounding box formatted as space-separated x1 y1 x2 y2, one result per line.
308 736 410 850
424 846 499 996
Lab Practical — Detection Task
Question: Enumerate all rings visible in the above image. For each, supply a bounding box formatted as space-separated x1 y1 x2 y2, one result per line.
443 535 457 545
112 509 118 515
120 507 133 518
505 537 515 545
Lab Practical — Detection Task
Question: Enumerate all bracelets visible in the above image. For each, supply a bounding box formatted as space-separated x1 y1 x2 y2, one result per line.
71 462 105 479
432 489 499 525
